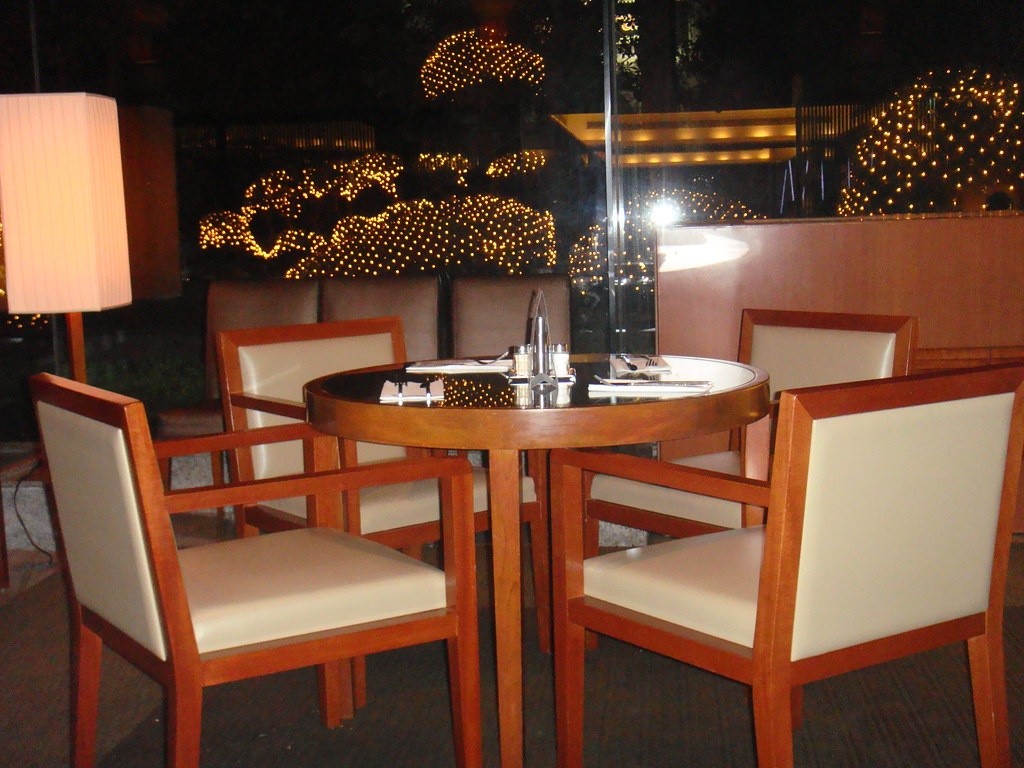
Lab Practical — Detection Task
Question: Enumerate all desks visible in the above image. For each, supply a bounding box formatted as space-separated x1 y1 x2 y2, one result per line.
303 354 770 768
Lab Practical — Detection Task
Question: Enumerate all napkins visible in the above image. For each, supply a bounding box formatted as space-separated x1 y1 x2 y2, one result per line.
379 379 444 403
588 379 712 393
406 359 513 374
610 354 671 372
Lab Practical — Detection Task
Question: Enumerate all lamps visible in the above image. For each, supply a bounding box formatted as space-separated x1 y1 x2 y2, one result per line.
0 93 133 586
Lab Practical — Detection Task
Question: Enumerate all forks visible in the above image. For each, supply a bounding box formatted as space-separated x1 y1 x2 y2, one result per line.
395 372 407 407
640 354 659 366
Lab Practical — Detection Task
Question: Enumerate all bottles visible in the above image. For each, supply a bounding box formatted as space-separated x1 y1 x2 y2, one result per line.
513 345 530 377
550 343 569 376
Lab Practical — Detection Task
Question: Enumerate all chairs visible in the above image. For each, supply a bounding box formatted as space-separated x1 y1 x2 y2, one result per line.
30 373 479 768
582 308 918 650
216 315 551 709
551 363 1024 768
